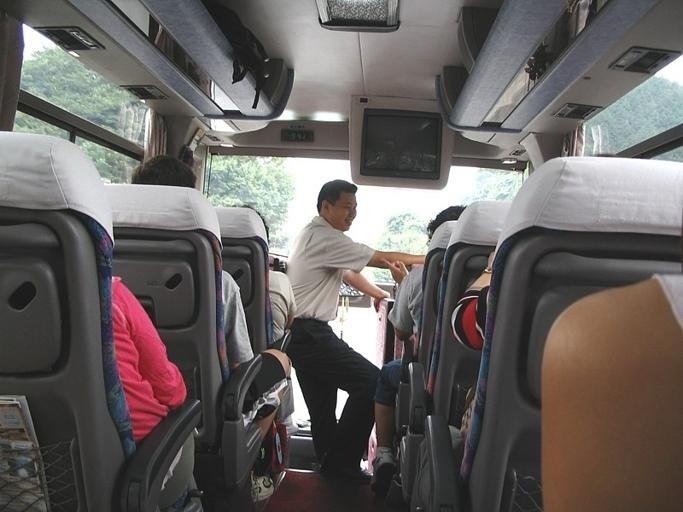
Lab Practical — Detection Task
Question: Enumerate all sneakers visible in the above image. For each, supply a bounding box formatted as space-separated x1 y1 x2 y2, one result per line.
249 471 274 500
369 453 396 481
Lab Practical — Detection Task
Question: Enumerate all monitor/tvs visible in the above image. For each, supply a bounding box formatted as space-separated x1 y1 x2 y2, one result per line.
349 95 455 190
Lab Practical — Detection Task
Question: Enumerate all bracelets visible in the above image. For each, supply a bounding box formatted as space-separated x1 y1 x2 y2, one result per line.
480 266 492 275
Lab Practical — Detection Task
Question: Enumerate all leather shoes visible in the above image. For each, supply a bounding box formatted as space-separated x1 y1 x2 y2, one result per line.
318 459 372 482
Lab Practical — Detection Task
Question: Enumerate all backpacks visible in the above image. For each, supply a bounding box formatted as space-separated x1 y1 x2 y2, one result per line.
208 0 273 110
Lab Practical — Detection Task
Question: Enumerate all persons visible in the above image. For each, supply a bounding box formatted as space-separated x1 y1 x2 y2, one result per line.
284 176 427 491
371 203 466 498
448 226 501 447
129 152 292 504
109 273 206 512
230 200 298 351
537 268 683 512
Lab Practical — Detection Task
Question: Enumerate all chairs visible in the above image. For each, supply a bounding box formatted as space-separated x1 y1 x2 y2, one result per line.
1 133 208 511
212 205 292 491
398 154 682 511
102 184 264 509
